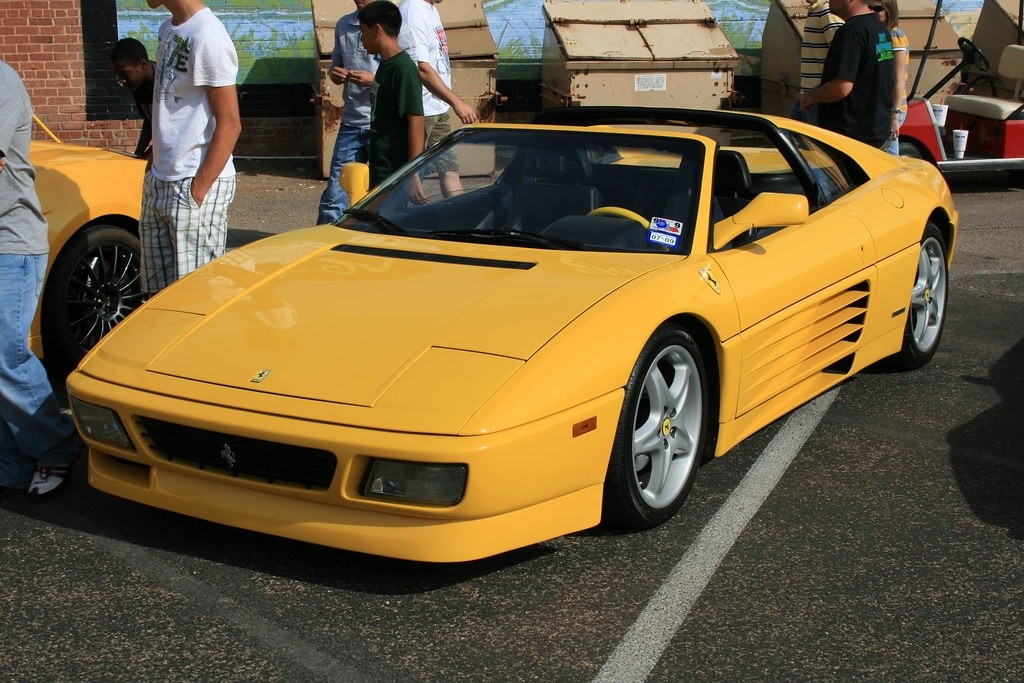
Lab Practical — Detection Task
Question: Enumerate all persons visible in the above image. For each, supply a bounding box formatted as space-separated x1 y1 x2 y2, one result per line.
0 59 80 503
109 37 156 160
789 0 909 156
139 0 244 300
316 0 478 225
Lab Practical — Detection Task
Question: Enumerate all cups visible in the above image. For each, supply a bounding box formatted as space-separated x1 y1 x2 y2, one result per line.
933 103 948 126
953 129 969 159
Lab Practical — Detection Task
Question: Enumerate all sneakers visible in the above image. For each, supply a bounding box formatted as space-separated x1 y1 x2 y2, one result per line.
26 449 83 504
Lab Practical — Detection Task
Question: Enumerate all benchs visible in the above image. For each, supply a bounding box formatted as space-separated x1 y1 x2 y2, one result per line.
945 44 1024 120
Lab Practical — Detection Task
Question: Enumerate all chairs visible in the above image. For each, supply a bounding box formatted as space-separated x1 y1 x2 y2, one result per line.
665 149 758 255
472 138 599 240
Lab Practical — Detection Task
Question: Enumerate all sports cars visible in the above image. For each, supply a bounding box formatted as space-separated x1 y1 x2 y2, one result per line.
26 113 152 383
65 96 962 566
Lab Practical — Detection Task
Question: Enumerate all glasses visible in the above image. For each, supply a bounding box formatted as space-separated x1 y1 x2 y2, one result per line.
867 5 885 12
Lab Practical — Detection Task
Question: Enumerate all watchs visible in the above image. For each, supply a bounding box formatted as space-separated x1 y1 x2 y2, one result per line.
891 108 902 114
